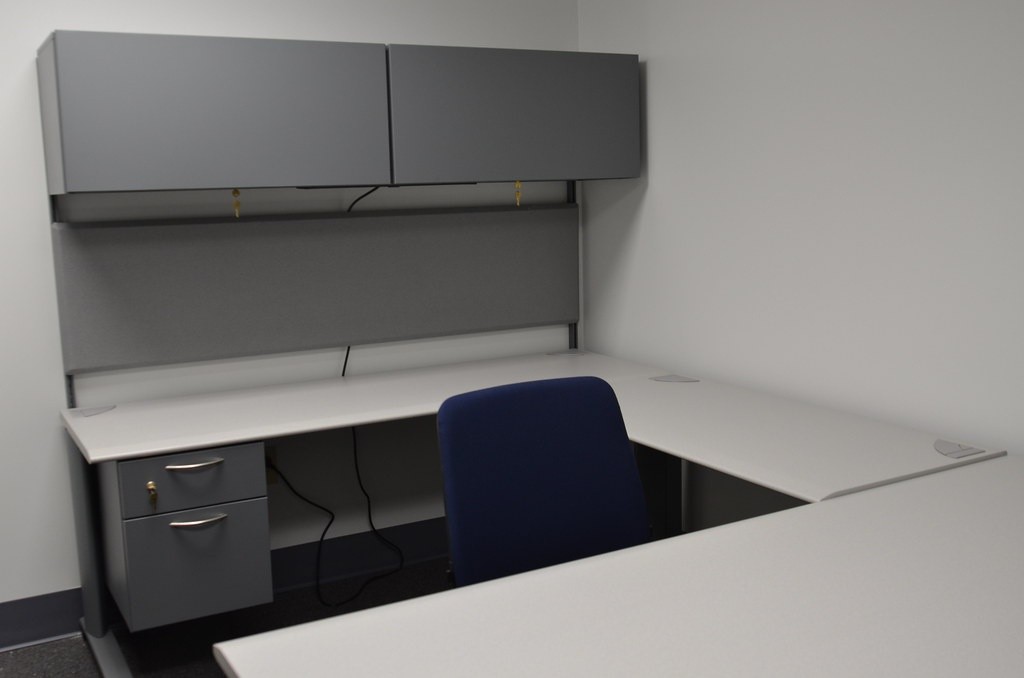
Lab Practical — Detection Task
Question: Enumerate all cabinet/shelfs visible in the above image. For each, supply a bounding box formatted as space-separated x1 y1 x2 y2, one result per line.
34 31 642 225
96 441 274 630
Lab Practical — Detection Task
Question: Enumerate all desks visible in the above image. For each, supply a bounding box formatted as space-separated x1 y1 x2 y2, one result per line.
62 350 1024 678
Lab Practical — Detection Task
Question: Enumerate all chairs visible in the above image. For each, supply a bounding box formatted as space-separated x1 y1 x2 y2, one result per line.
432 378 658 585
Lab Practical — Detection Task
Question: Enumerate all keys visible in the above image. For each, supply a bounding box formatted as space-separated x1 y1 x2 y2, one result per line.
231 189 242 218
511 180 525 207
